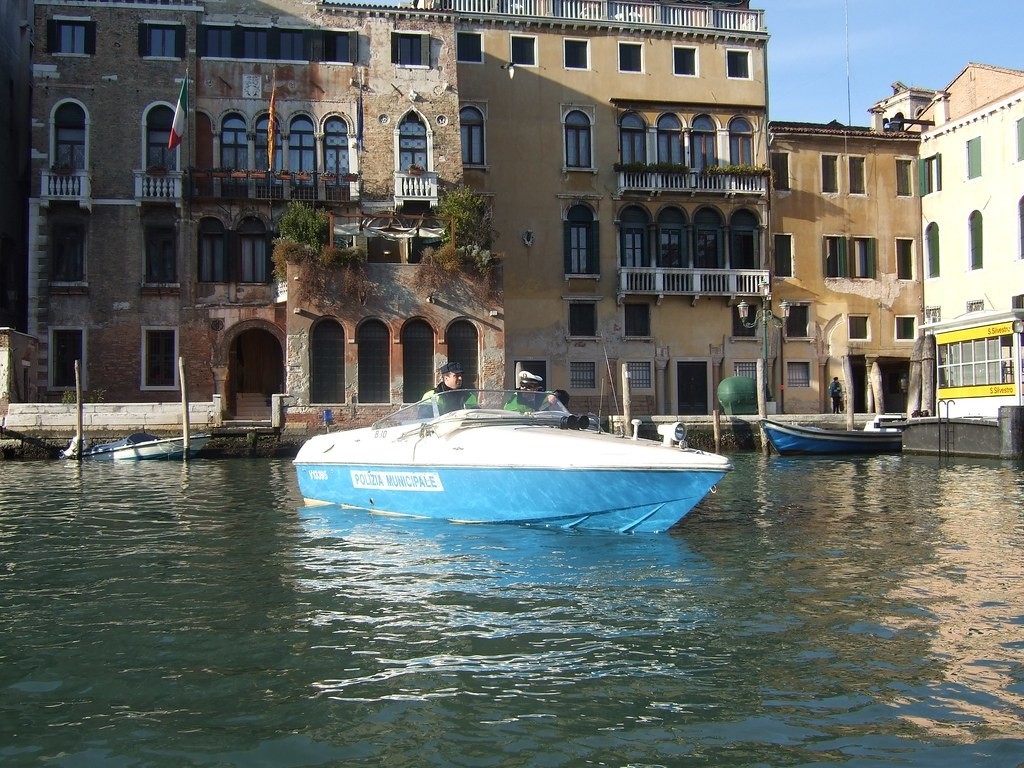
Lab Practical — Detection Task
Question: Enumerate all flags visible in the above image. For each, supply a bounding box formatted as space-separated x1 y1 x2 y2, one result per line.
168 73 188 150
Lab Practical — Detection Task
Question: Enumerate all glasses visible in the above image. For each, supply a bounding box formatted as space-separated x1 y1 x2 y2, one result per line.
521 383 534 389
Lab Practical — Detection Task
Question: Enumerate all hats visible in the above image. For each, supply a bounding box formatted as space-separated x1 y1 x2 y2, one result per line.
441 362 464 374
518 371 543 385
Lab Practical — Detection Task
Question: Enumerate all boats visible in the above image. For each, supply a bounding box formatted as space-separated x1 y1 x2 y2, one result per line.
759 414 906 455
293 386 733 536
61 431 213 462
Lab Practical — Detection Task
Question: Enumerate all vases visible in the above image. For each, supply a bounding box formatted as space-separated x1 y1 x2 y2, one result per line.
409 170 422 175
146 171 357 181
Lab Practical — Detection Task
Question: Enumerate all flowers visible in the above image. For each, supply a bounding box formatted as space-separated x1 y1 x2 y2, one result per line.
408 163 423 170
149 163 358 176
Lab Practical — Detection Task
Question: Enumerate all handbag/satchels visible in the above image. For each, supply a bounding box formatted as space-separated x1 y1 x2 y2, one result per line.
840 401 844 411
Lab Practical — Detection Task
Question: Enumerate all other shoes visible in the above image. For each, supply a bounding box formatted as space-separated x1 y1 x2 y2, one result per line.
837 412 839 413
833 411 835 413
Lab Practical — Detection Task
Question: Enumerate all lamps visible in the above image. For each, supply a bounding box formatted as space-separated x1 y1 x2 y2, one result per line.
883 119 935 130
522 230 535 247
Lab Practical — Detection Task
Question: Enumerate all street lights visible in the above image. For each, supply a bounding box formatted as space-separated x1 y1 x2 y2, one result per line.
737 280 791 402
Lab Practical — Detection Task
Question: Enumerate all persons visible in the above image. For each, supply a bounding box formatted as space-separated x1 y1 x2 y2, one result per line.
829 377 844 414
503 370 570 413
418 361 481 419
